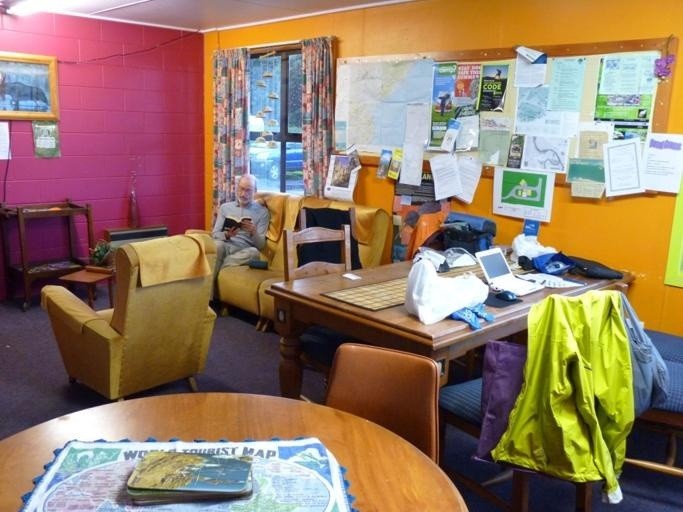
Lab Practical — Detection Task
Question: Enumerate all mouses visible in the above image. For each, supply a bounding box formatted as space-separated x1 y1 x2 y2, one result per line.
496 290 516 302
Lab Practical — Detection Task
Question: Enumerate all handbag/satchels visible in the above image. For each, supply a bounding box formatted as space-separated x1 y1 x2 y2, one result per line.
442 219 480 253
475 339 528 459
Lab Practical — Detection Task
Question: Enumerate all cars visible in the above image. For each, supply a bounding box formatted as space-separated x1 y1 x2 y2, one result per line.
248 128 302 180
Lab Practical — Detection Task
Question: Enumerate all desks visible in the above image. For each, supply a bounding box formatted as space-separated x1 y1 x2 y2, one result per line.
264 258 617 399
0 391 471 512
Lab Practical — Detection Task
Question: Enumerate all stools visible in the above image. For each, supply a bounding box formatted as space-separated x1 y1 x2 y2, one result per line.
58 266 114 311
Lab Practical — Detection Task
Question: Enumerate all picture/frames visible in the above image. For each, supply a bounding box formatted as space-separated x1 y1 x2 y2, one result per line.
0 51 62 121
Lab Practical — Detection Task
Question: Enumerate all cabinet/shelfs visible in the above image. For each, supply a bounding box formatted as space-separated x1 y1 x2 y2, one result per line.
0 196 100 311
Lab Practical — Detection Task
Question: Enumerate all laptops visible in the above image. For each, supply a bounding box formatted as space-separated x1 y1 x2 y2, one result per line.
475 247 543 297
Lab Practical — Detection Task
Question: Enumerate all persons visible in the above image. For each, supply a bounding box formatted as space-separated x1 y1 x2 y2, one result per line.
209 174 271 302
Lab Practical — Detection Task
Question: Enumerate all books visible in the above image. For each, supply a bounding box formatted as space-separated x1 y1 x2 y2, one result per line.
222 216 252 231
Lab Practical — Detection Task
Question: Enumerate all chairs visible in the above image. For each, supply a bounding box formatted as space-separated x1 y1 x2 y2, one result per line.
280 224 353 405
37 235 219 403
184 192 392 334
